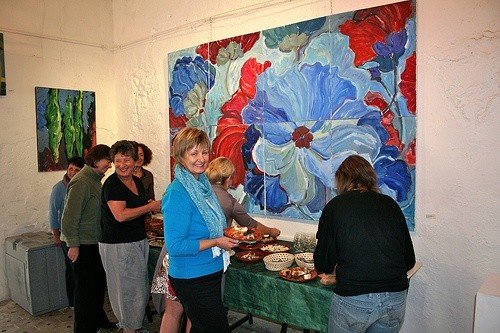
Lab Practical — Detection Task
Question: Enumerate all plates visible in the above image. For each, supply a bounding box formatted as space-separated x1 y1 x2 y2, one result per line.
237 241 263 250
146 230 164 238
223 226 263 241
280 267 318 281
148 237 165 247
260 244 290 254
235 251 264 263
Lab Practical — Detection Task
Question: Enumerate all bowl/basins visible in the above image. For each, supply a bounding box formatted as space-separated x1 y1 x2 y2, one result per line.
320 282 337 288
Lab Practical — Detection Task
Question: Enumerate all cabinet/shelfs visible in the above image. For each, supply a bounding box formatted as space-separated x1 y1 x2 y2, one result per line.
4 230 69 317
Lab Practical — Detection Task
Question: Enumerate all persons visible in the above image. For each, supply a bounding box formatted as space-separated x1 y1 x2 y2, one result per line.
60 144 118 333
98 140 161 333
132 143 155 203
151 157 280 333
49 157 85 310
313 155 415 333
161 127 240 333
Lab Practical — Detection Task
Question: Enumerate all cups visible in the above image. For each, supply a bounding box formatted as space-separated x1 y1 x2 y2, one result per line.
305 233 317 252
293 232 306 252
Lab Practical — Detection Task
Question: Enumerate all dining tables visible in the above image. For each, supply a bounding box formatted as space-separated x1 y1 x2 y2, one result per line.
144 239 335 333
